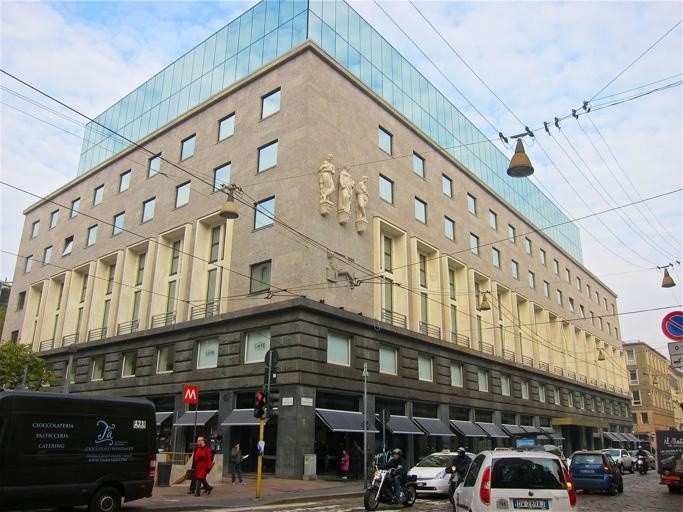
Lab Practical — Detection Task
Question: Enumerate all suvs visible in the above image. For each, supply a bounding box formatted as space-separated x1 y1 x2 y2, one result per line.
511 443 567 468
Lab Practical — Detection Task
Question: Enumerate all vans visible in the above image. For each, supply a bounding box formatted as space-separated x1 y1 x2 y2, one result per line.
0 390 156 510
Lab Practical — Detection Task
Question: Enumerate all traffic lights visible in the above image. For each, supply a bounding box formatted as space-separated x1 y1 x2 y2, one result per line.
267 387 279 416
253 391 264 418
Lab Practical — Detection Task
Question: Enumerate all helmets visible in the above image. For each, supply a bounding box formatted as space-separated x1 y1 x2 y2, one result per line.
458 448 465 455
393 448 401 455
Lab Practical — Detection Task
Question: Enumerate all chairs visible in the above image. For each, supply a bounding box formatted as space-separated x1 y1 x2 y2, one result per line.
578 456 602 464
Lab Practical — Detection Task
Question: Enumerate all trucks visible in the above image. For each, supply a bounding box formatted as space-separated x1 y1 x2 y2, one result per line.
654 429 683 494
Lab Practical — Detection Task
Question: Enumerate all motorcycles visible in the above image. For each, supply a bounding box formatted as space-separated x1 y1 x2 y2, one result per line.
363 461 417 510
635 455 648 475
445 464 464 503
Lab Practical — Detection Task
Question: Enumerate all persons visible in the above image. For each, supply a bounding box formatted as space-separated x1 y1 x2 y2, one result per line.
187 436 208 493
452 446 472 477
356 175 370 220
338 163 355 211
377 448 407 502
635 445 647 456
319 153 336 201
231 440 244 486
194 438 213 496
340 450 349 479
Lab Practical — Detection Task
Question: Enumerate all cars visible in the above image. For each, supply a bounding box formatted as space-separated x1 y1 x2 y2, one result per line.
626 449 656 470
566 449 585 471
600 447 633 474
406 448 476 496
450 448 577 512
566 449 623 495
